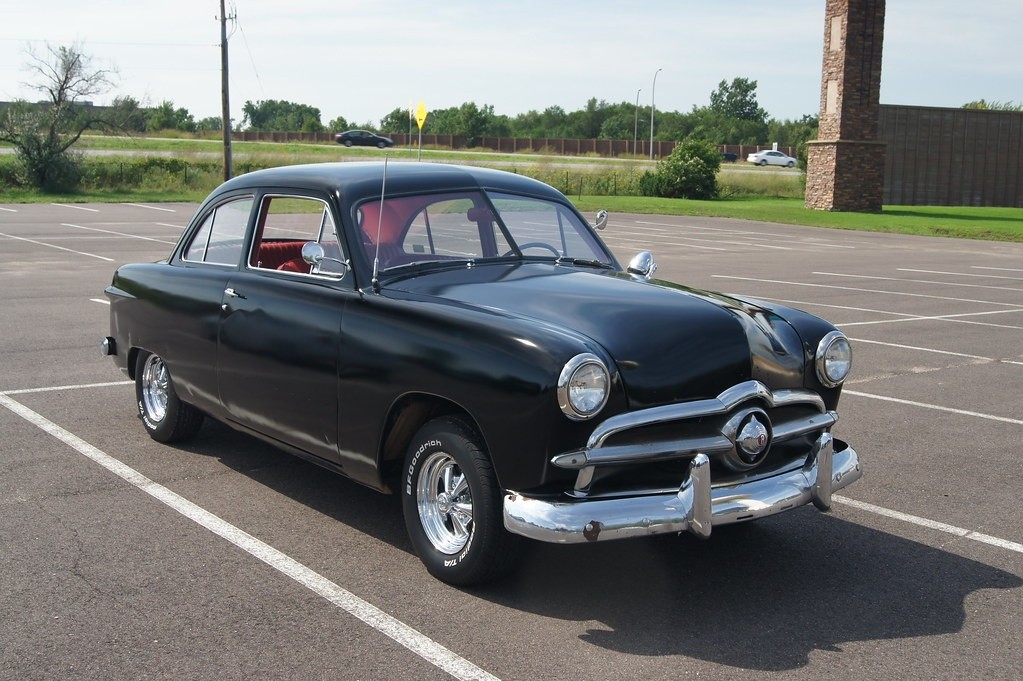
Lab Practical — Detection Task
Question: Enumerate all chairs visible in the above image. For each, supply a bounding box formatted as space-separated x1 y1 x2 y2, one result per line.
258 241 342 274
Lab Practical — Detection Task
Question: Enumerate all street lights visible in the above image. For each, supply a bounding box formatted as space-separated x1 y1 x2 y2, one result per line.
649 68 664 159
634 88 643 157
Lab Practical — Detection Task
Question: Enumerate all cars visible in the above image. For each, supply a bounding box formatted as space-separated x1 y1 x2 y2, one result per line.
334 130 394 148
746 149 798 168
101 153 866 593
